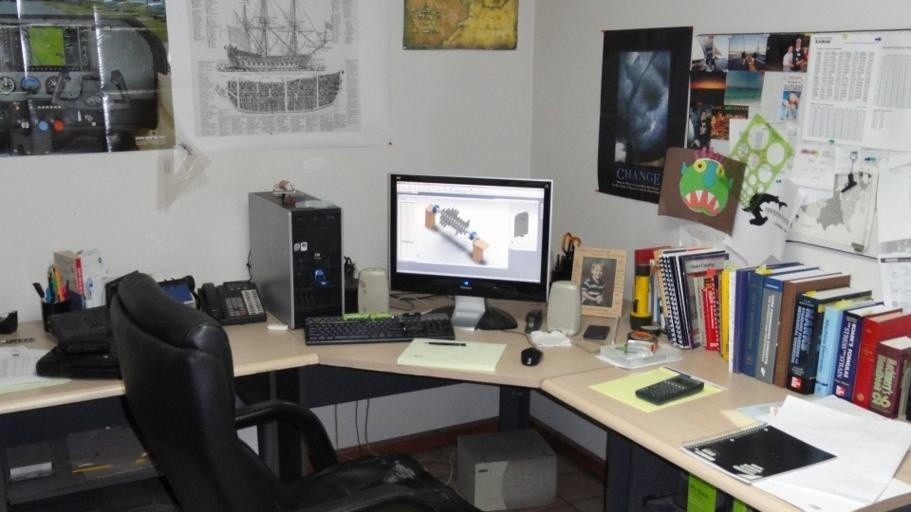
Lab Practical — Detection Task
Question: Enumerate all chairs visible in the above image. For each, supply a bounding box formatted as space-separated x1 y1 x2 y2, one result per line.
104 271 451 512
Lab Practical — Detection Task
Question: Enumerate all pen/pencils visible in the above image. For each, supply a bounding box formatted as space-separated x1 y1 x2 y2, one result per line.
423 341 467 347
33 265 69 304
612 318 621 344
555 254 566 273
1 337 36 344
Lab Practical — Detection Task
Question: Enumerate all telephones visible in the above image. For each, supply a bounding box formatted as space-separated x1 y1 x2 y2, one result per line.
198 280 267 325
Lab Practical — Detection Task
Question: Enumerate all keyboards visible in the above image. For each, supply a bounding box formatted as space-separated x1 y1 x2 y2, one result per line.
300 313 455 347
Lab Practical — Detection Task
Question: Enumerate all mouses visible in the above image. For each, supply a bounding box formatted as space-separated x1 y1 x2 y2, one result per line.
520 348 543 366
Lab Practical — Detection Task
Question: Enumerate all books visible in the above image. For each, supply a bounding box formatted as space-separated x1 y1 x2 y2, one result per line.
684 423 837 484
631 244 804 380
741 266 911 423
396 338 507 375
54 248 106 311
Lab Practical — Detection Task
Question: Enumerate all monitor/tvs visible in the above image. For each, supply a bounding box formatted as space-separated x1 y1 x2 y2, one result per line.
389 175 555 333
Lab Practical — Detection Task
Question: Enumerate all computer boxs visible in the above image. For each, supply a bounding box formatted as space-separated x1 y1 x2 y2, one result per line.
249 191 345 331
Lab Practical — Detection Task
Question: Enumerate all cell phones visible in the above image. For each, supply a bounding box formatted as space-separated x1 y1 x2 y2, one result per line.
583 323 610 343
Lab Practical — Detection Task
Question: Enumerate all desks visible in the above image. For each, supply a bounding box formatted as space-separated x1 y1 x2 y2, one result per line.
263 267 708 433
0 270 321 511
539 350 911 512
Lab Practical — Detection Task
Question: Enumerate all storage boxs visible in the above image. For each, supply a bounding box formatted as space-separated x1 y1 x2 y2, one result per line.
455 427 557 512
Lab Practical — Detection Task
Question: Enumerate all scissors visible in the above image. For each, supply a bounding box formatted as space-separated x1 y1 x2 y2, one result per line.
562 233 581 280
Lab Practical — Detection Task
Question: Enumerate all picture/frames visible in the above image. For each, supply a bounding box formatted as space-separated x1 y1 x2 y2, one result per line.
570 248 625 317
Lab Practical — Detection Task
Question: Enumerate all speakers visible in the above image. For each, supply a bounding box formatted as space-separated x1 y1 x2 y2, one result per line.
547 280 581 335
358 267 391 317
